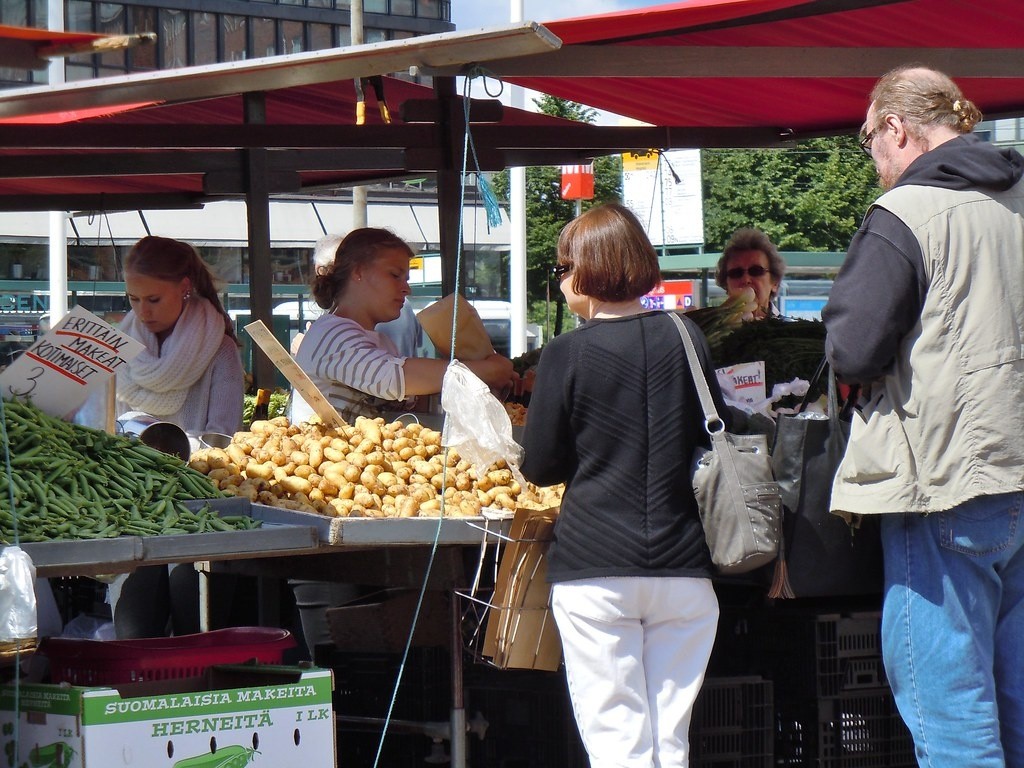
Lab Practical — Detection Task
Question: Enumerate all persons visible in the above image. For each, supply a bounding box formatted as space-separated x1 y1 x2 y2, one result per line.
518 205 720 768
288 228 521 768
291 235 423 359
113 236 245 642
715 228 786 321
822 65 1024 768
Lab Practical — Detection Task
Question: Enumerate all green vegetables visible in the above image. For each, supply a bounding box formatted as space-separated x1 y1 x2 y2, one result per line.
711 306 827 408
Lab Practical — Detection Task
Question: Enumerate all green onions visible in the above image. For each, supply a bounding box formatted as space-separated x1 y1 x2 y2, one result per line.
682 286 758 349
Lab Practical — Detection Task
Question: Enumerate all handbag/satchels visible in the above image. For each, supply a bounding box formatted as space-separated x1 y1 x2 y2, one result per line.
666 311 781 573
763 361 883 595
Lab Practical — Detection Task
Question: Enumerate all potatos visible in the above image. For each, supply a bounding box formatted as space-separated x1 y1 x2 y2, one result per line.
186 417 567 518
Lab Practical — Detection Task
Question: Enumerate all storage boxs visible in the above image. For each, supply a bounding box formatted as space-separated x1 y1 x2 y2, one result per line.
464 610 914 768
1 661 336 768
40 625 298 682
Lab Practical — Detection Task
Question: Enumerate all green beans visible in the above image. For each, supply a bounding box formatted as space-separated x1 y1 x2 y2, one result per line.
0 392 265 546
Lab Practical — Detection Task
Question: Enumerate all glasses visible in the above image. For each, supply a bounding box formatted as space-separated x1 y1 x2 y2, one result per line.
859 121 884 158
724 265 770 279
552 263 571 281
